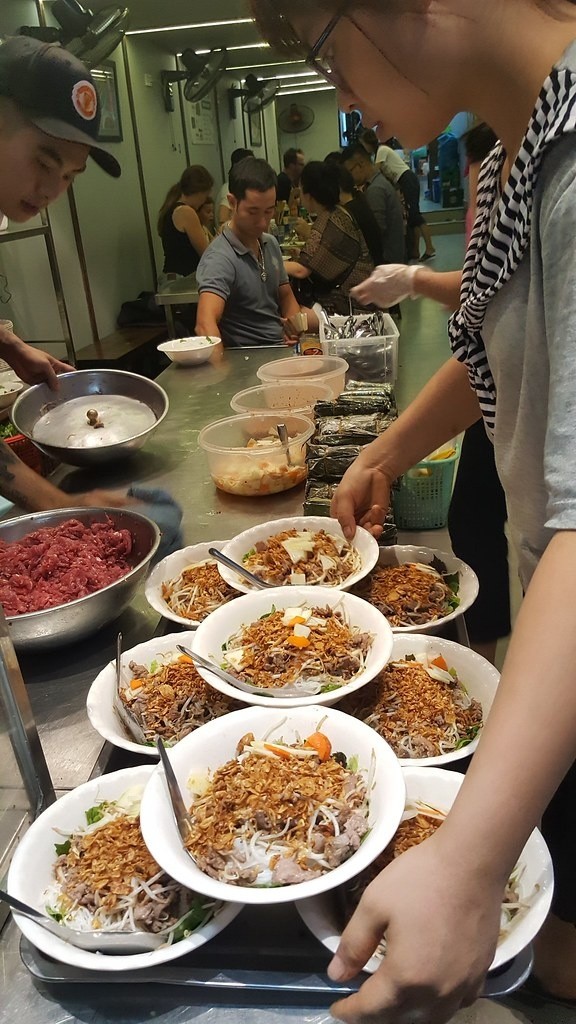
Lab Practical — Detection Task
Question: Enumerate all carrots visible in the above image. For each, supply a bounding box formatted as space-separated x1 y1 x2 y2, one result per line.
431 656 448 671
288 616 307 625
287 635 310 648
263 744 290 759
177 654 192 665
304 732 331 762
130 680 143 690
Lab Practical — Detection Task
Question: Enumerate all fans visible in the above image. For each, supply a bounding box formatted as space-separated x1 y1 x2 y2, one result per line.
21 1 131 68
277 104 315 132
159 41 228 113
228 74 278 122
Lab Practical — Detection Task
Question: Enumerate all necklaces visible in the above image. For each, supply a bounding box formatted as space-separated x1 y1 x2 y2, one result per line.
228 222 267 282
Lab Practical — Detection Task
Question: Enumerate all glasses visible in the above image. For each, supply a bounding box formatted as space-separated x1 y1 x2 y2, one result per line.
306 0 347 93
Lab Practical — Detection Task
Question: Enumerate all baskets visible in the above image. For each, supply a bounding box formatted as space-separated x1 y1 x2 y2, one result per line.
390 443 460 530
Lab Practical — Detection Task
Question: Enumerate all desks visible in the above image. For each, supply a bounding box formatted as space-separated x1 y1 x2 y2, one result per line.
0 345 306 788
155 221 296 340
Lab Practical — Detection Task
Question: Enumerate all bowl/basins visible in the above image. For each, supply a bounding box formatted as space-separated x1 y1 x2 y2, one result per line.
9 368 170 467
196 355 349 497
157 335 223 366
0 382 23 409
5 515 557 973
0 319 32 393
0 505 160 651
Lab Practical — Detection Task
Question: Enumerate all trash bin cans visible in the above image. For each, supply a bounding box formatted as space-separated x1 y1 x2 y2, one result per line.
432 179 441 203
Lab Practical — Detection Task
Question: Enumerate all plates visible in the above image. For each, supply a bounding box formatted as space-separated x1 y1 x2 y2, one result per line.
282 256 291 261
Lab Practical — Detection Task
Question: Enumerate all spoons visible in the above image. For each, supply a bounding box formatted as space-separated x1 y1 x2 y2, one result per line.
324 312 386 338
0 890 164 954
114 632 148 746
175 645 314 698
206 547 318 589
153 734 199 864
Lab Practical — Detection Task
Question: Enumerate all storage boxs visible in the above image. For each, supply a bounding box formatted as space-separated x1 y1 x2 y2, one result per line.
319 313 400 385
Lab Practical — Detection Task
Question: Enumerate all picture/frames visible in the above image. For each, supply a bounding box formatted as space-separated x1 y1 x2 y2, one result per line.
93 60 124 143
248 102 263 147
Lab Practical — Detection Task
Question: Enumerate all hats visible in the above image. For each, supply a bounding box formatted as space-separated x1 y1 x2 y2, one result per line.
0 35 120 178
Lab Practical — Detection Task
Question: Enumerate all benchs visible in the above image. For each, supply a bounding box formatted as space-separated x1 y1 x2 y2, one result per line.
58 311 170 363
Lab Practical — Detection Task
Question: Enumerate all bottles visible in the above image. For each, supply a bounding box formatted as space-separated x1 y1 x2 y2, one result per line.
268 218 279 242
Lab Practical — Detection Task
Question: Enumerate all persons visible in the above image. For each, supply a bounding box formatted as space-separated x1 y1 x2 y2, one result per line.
0 36 148 512
215 148 254 228
248 0 575 1024
197 198 223 242
158 165 215 295
277 149 305 206
359 128 435 263
324 144 404 264
330 126 510 667
332 165 382 266
195 158 301 346
284 161 374 316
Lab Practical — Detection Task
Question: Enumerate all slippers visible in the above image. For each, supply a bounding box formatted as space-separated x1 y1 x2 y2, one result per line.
418 254 436 263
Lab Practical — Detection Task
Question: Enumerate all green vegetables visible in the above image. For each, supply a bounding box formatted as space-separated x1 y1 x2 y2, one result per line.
0 417 19 439
